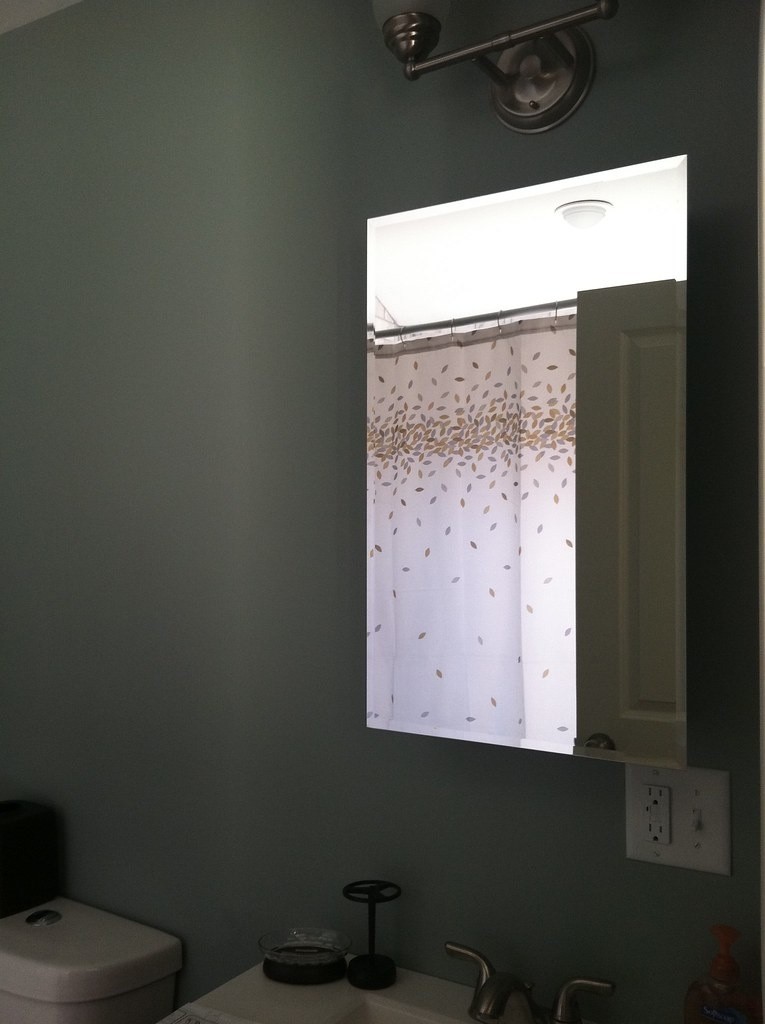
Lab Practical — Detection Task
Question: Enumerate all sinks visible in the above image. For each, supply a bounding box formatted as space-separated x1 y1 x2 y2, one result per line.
314 991 477 1024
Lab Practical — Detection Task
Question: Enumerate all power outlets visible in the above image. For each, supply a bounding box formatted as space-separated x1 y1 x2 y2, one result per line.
625 764 735 875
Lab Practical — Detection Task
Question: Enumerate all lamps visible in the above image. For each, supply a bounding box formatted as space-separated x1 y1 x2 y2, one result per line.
369 0 625 135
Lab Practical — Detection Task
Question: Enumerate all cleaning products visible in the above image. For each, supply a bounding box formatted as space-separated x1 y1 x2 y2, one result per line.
683 923 763 1024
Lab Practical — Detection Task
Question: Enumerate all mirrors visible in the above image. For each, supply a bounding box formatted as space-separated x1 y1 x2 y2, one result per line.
361 148 691 765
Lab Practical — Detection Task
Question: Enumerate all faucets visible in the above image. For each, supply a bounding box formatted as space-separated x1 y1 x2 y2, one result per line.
468 971 551 1024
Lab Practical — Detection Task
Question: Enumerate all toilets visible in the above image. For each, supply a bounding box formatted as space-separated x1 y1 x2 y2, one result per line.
0 895 184 1024
